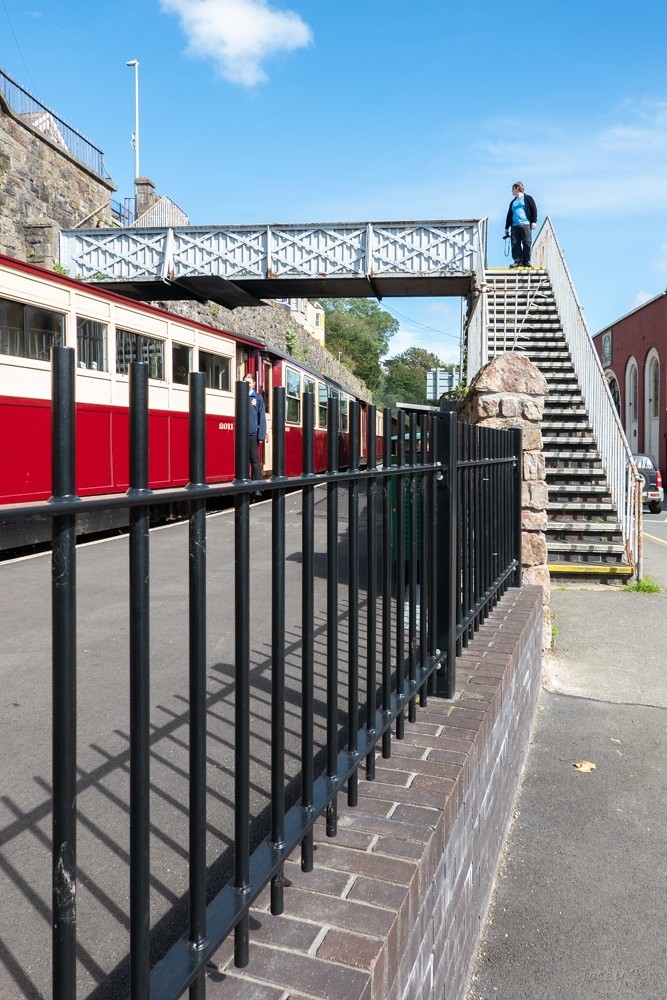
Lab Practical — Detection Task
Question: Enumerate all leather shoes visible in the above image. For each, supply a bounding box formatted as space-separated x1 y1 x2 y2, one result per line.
255 491 261 496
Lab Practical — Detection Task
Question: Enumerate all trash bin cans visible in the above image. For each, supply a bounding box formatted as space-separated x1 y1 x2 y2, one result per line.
392 433 430 562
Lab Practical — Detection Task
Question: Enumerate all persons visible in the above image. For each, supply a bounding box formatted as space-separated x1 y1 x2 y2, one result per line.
241 372 267 496
504 182 537 268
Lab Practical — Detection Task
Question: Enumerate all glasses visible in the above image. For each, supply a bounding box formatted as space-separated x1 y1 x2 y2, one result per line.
513 187 515 190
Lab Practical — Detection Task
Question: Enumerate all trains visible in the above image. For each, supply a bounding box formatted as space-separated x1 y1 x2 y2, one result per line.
0 250 417 552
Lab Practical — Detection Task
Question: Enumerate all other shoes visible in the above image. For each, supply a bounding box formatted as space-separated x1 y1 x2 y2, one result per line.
510 261 523 267
523 261 530 266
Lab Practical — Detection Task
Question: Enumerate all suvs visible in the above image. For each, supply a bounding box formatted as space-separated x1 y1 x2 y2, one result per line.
626 453 664 514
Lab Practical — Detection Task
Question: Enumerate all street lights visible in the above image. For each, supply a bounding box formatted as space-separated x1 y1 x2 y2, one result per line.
126 58 141 219
